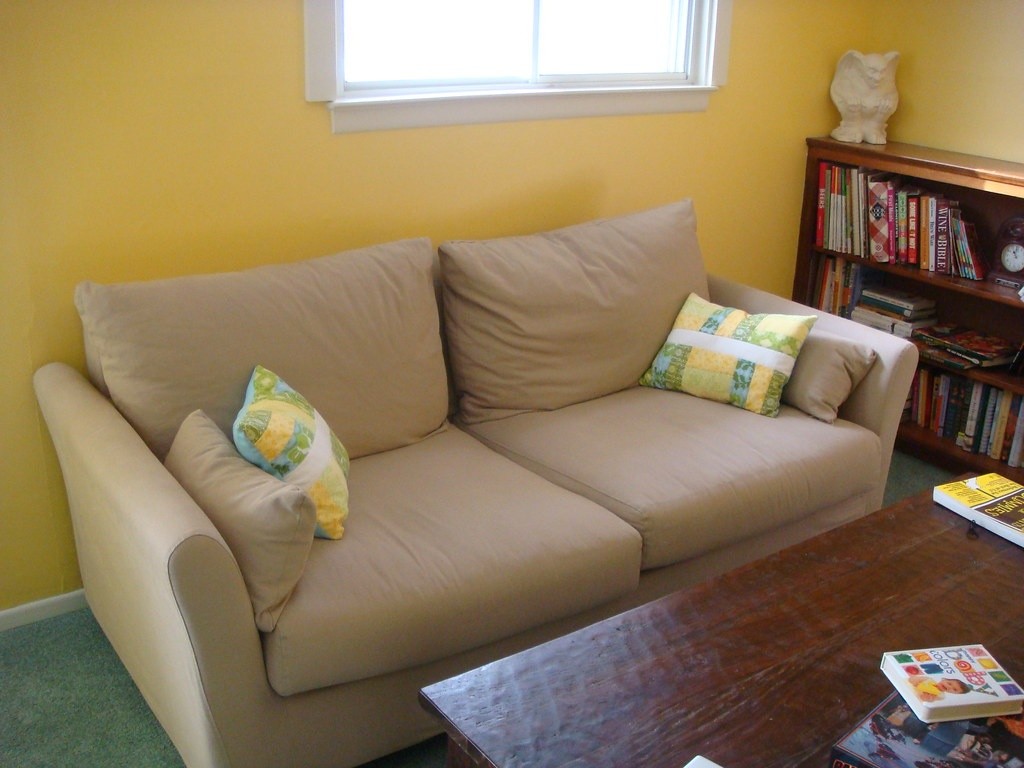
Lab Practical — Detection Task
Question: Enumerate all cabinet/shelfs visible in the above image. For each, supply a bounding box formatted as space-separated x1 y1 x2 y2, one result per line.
793 132 1024 480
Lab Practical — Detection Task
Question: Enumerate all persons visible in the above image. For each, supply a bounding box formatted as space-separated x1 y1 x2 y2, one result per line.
908 675 970 702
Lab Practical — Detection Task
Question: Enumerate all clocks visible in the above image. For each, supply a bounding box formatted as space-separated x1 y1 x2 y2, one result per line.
996 226 1024 290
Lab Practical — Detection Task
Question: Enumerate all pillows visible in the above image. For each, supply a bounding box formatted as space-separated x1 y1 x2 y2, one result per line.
159 364 353 631
75 237 450 458
437 200 711 424
640 290 877 424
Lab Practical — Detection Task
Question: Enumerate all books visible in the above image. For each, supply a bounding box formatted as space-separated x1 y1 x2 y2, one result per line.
814 160 987 281
933 473 1024 549
901 362 1024 468
829 644 1024 768
813 255 1023 369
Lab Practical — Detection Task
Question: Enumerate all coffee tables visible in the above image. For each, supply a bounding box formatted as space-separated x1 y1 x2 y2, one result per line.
417 468 1024 768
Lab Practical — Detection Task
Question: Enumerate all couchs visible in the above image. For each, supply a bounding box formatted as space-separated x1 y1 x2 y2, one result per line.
34 280 920 768
